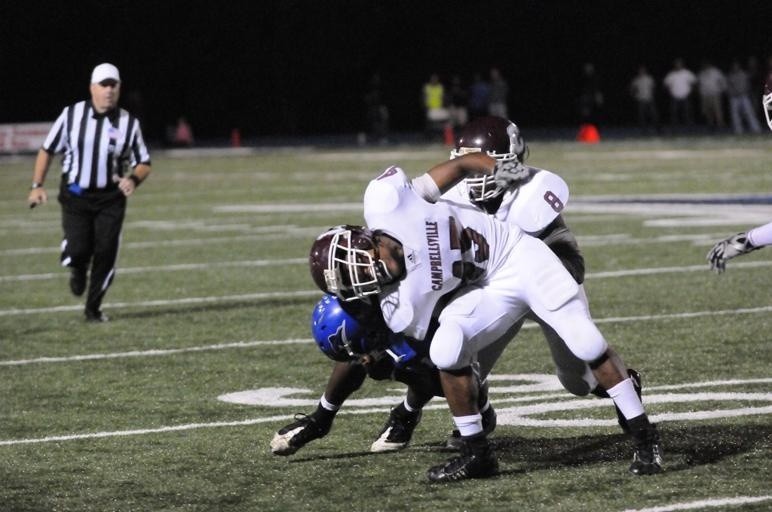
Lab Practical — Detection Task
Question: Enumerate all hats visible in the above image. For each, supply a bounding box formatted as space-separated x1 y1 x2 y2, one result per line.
91 63 122 84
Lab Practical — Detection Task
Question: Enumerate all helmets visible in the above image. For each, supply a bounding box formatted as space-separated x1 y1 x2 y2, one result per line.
311 225 394 305
312 294 390 366
449 118 528 202
762 72 771 132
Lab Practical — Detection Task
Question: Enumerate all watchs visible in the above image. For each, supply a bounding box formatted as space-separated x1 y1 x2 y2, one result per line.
31 183 41 189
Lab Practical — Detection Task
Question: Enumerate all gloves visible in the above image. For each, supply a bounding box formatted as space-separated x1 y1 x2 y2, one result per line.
707 231 755 275
495 161 530 187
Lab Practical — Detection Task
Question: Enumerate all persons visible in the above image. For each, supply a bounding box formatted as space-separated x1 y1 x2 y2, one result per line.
698 57 729 131
748 58 763 114
29 62 152 322
663 55 698 133
487 70 510 120
311 154 662 482
726 62 762 136
312 215 641 449
449 77 468 134
270 116 570 456
706 71 772 275
422 74 449 137
631 66 659 137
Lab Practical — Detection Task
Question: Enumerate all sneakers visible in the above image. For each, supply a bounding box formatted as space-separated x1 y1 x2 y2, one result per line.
632 423 665 473
270 404 333 457
70 264 86 296
615 368 642 435
428 446 502 482
370 404 422 452
83 305 112 323
446 407 497 452
